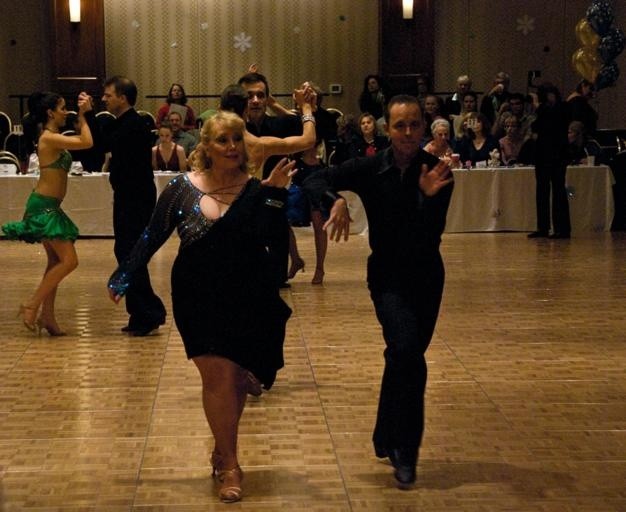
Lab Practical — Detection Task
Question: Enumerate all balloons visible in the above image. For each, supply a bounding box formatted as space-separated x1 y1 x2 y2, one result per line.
571 0 626 91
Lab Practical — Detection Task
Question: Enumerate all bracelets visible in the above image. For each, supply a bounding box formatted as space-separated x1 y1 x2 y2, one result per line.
301 114 316 125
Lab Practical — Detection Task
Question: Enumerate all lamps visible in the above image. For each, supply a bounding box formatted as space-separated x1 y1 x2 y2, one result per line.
68 0 81 22
403 0 413 19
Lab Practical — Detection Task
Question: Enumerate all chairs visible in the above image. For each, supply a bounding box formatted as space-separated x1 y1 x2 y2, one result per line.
0 111 159 174
289 108 344 167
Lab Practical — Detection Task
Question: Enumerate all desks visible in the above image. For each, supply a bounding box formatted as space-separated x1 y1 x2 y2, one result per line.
0 165 616 236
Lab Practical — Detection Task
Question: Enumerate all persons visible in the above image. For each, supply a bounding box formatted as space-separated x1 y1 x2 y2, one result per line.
417 71 600 167
249 62 327 284
301 95 454 487
528 82 572 240
238 73 319 180
333 74 386 166
151 83 200 174
78 75 168 336
1 91 93 335
107 111 301 505
218 83 317 182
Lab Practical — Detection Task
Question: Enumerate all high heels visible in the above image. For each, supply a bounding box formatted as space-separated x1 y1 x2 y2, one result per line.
36 311 66 338
312 267 325 285
209 450 246 504
287 257 306 278
15 293 41 331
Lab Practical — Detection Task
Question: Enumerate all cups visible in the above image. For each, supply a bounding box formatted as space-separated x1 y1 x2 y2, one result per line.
497 83 504 94
450 153 460 168
586 156 596 165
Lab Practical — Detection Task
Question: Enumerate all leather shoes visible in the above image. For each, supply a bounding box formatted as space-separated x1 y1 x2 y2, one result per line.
526 229 572 239
384 445 417 489
121 318 165 337
